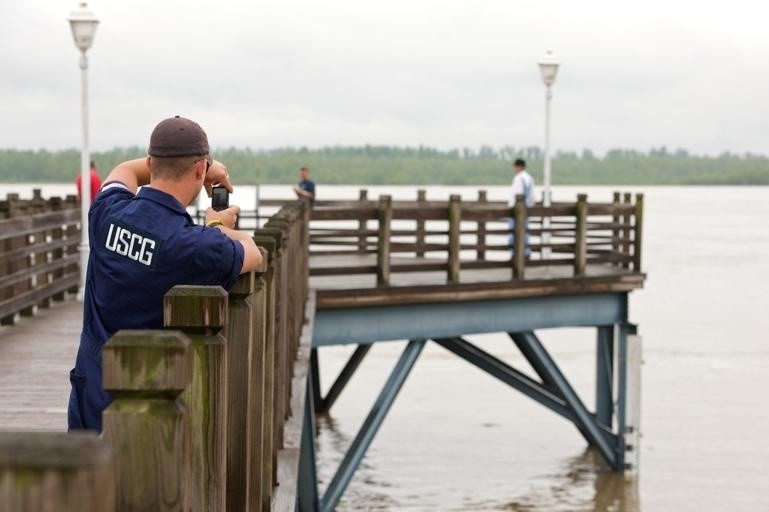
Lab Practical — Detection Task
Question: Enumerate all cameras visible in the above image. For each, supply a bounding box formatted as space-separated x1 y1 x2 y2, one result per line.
210 186 229 212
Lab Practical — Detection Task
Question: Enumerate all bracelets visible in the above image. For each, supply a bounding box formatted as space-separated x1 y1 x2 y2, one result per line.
205 219 222 229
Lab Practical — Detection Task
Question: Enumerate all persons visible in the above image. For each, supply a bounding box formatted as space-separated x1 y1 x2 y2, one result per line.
75 159 102 205
292 167 315 212
507 158 535 267
64 114 264 437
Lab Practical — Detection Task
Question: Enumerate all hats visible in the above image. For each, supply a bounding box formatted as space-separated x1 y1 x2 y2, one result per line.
148 115 210 157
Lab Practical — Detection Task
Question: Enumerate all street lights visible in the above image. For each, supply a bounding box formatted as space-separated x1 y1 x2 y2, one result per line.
538 51 559 260
69 3 99 303
